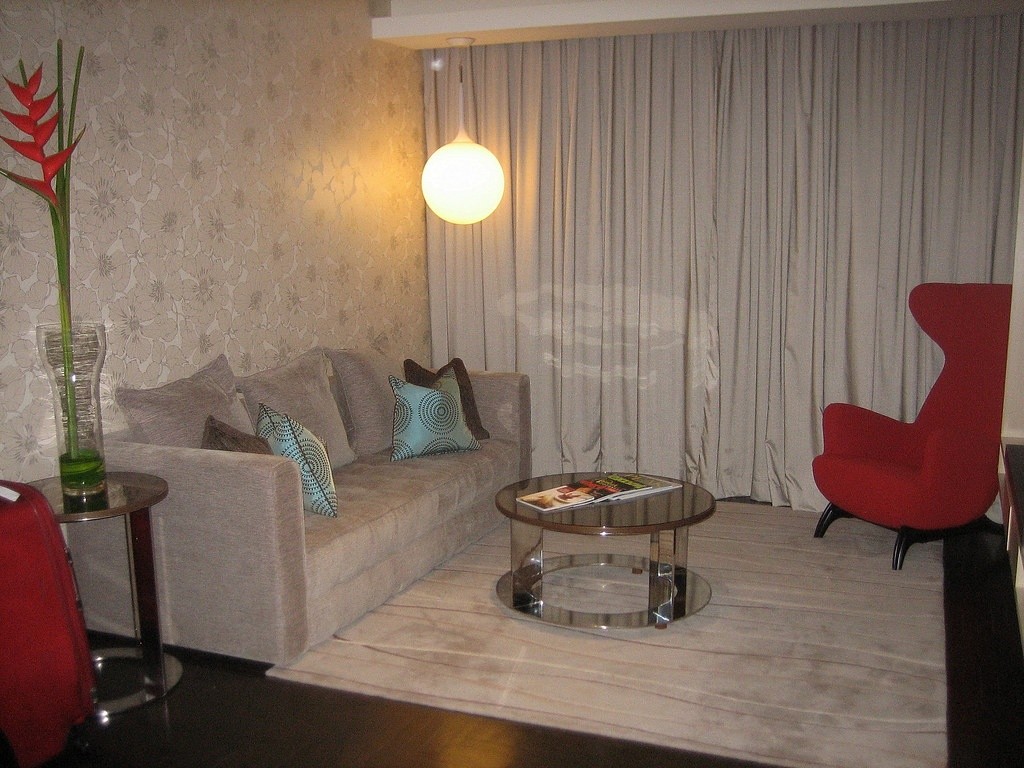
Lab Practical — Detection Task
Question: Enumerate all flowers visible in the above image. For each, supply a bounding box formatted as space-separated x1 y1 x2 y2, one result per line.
0 37 107 497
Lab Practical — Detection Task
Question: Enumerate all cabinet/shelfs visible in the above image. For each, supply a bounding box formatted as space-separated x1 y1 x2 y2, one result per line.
998 437 1024 655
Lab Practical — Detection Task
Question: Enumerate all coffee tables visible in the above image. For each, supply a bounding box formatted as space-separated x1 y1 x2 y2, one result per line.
496 471 717 630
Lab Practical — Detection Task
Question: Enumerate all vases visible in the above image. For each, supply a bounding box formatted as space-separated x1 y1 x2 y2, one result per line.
34 319 107 510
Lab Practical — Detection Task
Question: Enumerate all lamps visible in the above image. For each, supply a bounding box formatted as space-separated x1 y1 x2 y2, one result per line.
421 37 505 225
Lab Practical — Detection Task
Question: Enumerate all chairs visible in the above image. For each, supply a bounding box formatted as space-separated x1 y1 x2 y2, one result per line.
811 283 1012 570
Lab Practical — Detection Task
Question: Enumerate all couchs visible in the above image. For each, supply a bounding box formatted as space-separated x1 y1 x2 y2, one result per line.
67 372 532 666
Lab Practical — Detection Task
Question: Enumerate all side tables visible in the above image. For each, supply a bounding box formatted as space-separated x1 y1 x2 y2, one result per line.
25 472 185 716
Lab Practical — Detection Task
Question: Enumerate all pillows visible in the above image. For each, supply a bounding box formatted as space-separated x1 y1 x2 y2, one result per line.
114 355 256 449
235 346 358 471
201 416 273 456
323 330 405 462
255 402 338 518
404 358 490 442
389 368 482 461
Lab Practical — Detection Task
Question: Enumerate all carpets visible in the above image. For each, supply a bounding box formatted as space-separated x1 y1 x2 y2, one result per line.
264 500 947 768
